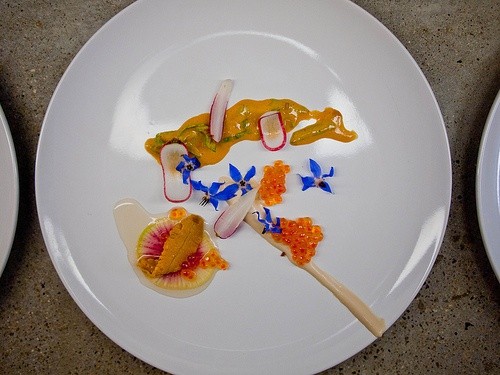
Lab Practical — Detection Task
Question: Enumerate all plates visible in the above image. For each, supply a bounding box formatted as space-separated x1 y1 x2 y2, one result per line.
0 103 20 279
34 0 453 375
475 87 500 283
